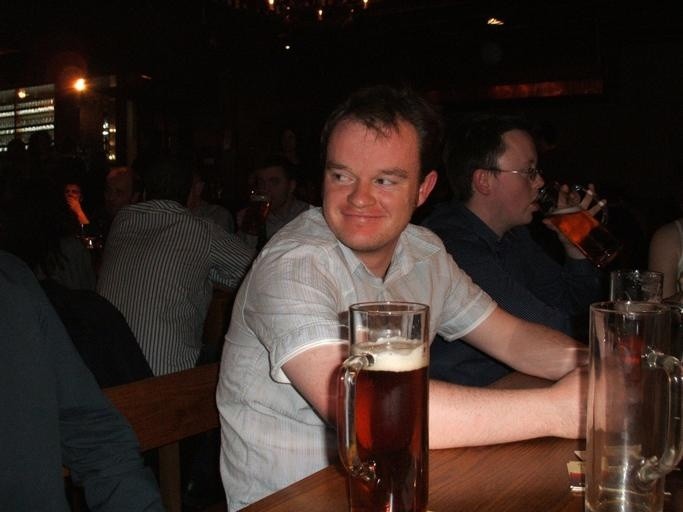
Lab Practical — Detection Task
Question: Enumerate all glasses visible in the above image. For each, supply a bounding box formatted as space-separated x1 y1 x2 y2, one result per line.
483 168 543 181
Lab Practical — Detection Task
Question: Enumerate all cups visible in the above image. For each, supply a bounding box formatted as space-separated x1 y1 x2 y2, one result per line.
532 183 626 270
583 299 682 508
609 267 664 308
240 189 271 238
334 299 432 508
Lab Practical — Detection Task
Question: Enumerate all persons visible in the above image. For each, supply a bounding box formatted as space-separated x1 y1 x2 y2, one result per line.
26 130 54 169
95 144 257 378
0 138 26 168
238 156 315 252
649 216 683 299
216 86 591 512
99 166 144 242
63 180 90 225
0 250 163 512
184 171 234 236
419 112 611 388
1 168 96 294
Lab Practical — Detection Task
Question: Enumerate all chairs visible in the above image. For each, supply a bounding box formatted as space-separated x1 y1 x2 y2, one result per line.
102 358 221 512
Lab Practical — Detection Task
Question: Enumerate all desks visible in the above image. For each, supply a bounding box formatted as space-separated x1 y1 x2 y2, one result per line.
233 289 683 511
